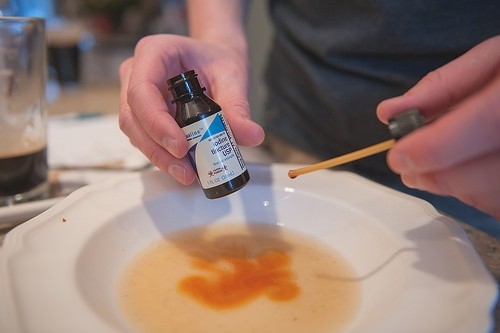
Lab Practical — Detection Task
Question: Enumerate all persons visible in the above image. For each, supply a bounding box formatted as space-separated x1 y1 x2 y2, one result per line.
119 0 500 221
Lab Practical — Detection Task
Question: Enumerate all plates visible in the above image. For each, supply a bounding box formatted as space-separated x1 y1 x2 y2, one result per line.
44 113 159 188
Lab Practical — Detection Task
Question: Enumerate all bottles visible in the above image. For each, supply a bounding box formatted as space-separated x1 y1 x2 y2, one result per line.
1 162 498 333
167 71 250 199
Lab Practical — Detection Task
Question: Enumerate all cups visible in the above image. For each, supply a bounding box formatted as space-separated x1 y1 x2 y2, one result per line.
0 17 49 204
